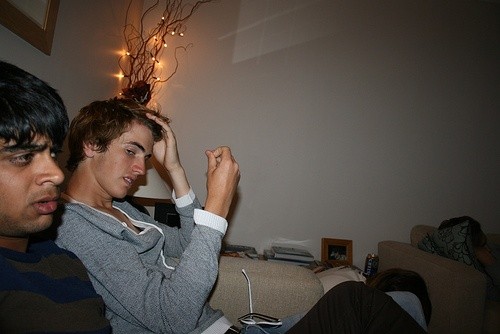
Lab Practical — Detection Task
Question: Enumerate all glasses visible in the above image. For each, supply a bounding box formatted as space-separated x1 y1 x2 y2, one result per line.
238 269 283 326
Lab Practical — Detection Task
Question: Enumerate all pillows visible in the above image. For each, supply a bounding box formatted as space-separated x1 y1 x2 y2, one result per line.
418 220 495 286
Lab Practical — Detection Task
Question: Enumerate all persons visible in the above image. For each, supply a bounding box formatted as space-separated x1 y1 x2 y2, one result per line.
316 264 432 326
50 97 427 334
0 61 427 334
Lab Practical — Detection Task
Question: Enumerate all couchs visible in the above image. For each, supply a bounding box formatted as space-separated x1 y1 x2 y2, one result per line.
208 256 324 327
378 224 500 334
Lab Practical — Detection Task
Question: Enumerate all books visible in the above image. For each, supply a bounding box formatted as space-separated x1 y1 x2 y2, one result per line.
223 245 259 259
264 246 314 266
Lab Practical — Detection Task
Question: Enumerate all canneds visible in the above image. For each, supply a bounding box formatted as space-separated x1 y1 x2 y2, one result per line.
366 254 378 276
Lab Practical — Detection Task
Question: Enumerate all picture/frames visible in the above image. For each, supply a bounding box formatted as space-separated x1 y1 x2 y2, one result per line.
0 0 61 56
321 238 353 267
